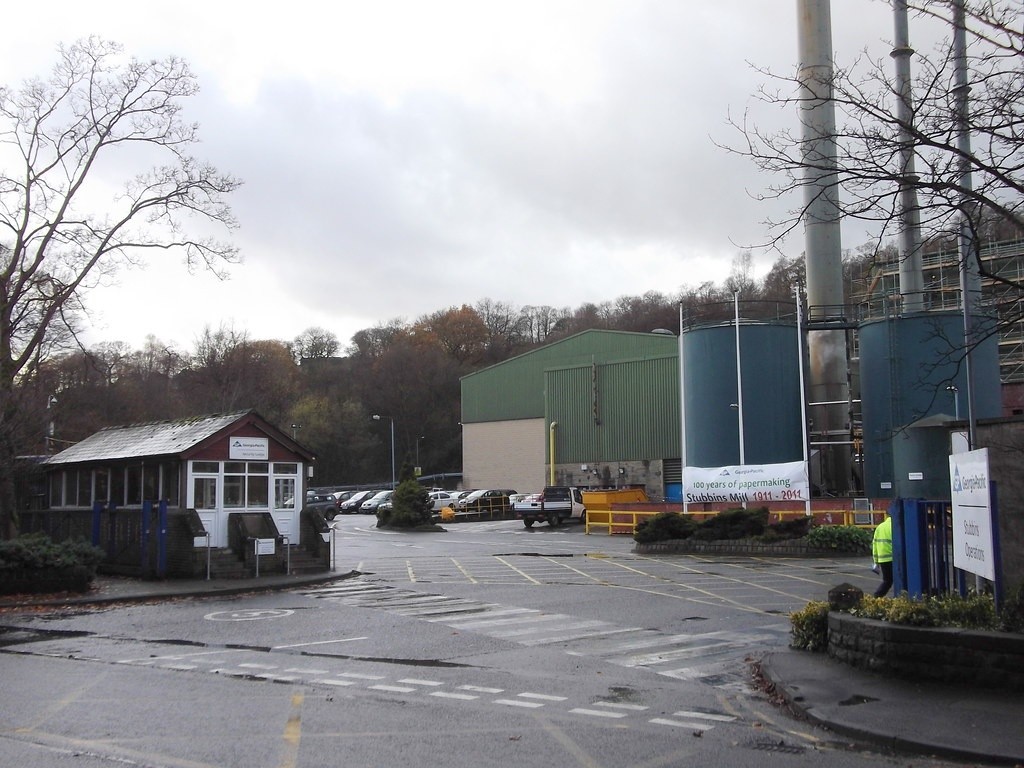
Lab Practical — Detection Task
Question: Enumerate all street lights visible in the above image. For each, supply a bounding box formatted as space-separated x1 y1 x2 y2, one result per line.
373 415 395 492
44 395 57 459
416 434 425 466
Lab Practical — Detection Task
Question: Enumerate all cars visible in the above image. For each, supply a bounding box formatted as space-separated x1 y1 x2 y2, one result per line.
450 490 473 504
308 490 337 496
361 490 397 514
333 491 362 511
428 491 462 514
458 490 509 512
340 490 384 515
377 500 394 514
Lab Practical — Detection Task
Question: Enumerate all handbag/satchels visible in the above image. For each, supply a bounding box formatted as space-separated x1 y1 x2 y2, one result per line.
871 564 881 575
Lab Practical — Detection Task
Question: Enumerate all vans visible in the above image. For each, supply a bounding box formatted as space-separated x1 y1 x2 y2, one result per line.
283 494 340 521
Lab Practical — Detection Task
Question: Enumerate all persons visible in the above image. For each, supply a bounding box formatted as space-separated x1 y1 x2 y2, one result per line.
873 508 893 598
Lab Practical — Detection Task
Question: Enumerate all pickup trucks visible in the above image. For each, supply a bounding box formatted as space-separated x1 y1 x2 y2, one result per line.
514 485 587 528
